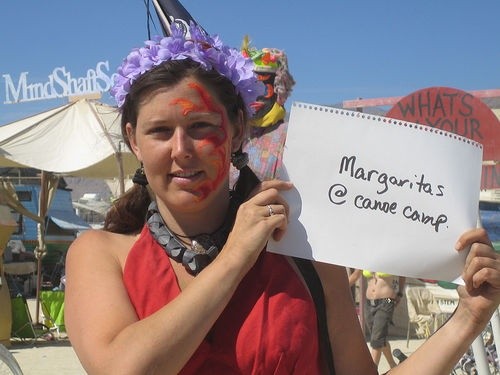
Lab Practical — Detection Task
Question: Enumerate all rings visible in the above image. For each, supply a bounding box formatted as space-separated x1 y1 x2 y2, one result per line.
266 203 274 217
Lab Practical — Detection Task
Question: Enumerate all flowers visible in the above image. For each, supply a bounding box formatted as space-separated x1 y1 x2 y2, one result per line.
107 20 266 118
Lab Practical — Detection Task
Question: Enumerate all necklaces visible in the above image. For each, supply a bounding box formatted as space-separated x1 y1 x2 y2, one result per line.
146 189 244 277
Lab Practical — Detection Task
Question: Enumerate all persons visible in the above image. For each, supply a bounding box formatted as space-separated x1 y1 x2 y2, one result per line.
63 18 500 375
349 267 407 369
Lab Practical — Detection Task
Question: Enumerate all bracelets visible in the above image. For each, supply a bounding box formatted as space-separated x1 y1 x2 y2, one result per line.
397 291 403 298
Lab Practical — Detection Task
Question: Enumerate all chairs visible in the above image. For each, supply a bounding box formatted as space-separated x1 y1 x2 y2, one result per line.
406 287 443 347
12 296 54 346
40 290 68 338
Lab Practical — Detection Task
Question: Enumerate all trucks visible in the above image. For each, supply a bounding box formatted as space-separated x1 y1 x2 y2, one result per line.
0 167 93 294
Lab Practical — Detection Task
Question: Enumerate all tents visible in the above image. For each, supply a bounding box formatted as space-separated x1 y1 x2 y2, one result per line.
0 96 141 325
